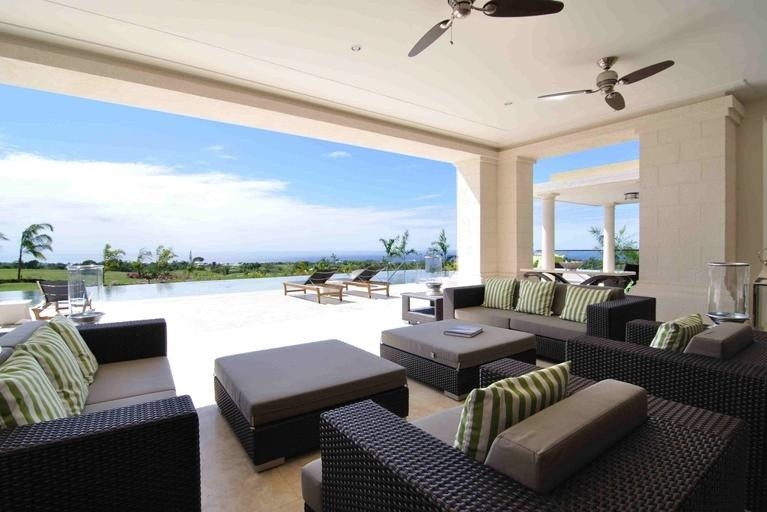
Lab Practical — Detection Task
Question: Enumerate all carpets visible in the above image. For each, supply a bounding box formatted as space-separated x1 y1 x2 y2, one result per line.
198 363 481 512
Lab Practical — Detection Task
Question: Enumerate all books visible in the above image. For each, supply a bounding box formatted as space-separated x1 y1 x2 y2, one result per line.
443 321 485 339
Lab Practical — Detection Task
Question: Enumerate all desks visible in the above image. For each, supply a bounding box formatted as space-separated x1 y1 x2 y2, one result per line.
399 291 442 324
581 267 635 287
519 268 569 283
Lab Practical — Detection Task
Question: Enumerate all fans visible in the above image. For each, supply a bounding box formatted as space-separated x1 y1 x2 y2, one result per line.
537 57 676 111
406 0 565 59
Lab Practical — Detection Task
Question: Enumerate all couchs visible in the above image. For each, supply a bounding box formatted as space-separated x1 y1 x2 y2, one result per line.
443 277 656 363
0 317 201 512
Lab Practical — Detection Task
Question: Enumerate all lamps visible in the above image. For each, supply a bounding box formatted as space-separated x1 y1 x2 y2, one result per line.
425 256 442 296
706 262 750 325
625 192 638 201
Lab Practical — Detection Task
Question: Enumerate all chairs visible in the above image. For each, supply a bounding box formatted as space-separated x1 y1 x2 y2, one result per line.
301 357 752 512
565 317 767 511
32 279 95 319
284 268 344 303
343 261 390 298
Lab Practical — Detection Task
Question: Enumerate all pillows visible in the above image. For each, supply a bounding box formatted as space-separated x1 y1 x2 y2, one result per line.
50 313 100 385
513 276 558 315
558 281 611 325
648 313 703 353
454 359 574 460
18 324 91 416
0 346 67 430
482 277 517 308
